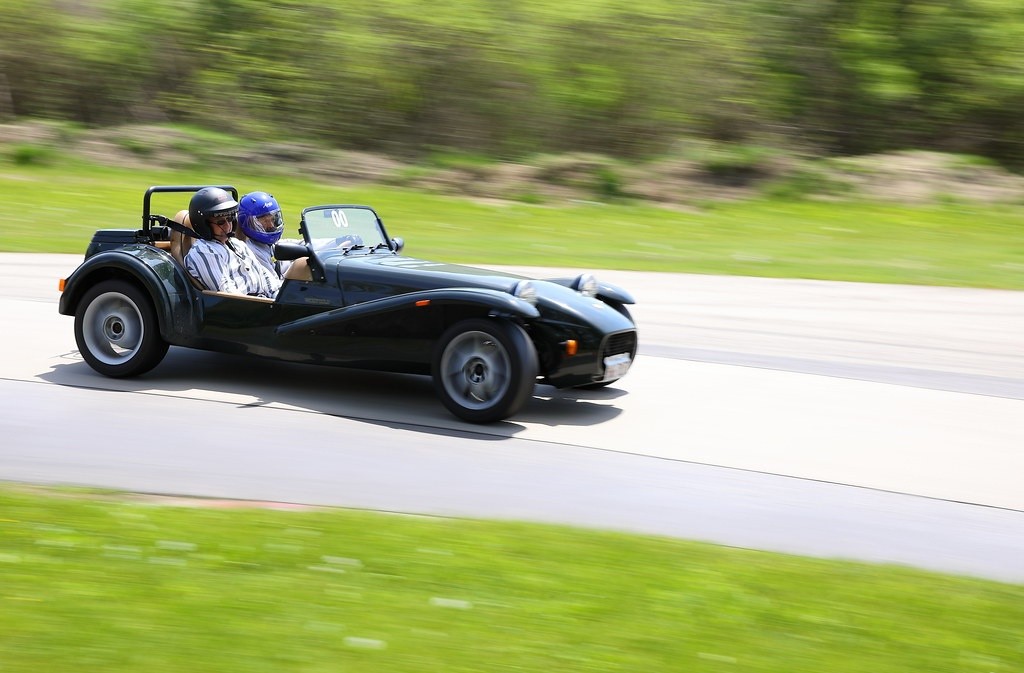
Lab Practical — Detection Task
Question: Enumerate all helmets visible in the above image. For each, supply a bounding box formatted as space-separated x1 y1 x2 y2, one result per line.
189 187 238 241
238 191 284 245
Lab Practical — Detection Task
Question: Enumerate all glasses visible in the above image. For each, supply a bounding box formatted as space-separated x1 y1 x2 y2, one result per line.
208 216 233 226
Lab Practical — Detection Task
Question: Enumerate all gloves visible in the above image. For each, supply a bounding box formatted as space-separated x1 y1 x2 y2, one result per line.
336 234 364 247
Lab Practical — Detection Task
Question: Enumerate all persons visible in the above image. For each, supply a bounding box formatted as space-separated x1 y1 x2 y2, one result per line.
184 187 364 300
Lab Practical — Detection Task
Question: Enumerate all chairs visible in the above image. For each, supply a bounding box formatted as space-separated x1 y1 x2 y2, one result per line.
172 211 206 292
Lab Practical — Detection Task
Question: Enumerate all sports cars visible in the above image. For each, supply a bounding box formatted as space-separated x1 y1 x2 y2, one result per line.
59 185 638 424
